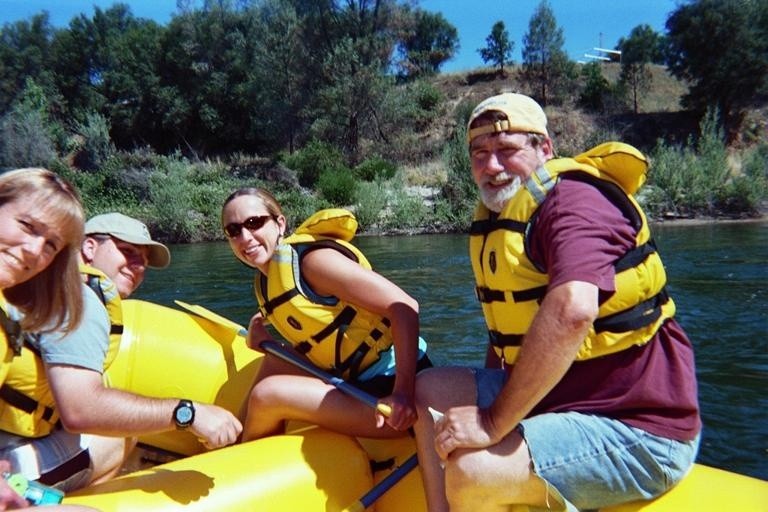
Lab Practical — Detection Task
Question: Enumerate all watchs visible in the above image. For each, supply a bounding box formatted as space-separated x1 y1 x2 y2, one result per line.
173 398 196 430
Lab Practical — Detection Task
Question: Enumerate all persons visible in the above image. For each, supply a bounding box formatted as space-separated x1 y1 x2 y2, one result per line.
409 94 704 512
0 212 243 492
222 186 434 442
0 166 87 512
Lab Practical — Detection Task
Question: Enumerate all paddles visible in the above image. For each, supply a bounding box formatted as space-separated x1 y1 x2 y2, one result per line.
173 299 393 418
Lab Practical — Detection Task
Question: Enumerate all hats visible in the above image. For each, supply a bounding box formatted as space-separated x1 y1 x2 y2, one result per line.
84 212 171 270
466 92 549 146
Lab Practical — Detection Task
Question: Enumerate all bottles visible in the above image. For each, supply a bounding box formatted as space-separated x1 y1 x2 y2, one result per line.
1 472 66 509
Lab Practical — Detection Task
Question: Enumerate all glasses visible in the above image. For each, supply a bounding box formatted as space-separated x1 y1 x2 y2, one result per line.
223 215 273 237
